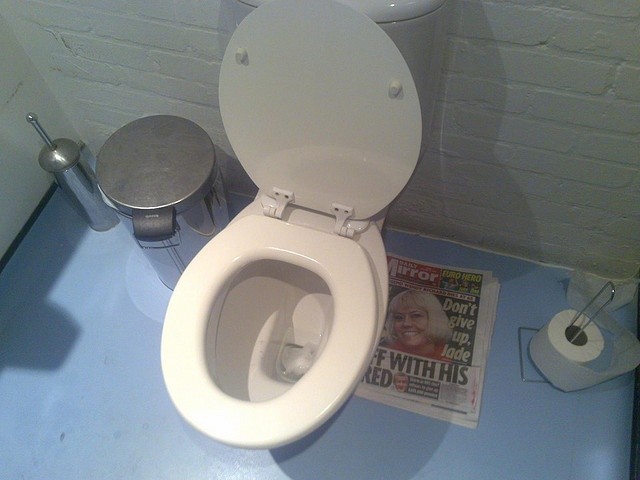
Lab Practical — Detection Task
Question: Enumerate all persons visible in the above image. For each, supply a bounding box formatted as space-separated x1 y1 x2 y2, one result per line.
383 289 454 361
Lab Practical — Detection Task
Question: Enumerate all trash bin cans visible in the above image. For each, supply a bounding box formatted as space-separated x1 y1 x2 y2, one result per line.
95 114 236 291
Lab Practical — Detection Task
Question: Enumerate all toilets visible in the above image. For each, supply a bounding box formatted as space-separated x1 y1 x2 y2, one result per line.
158 0 451 456
